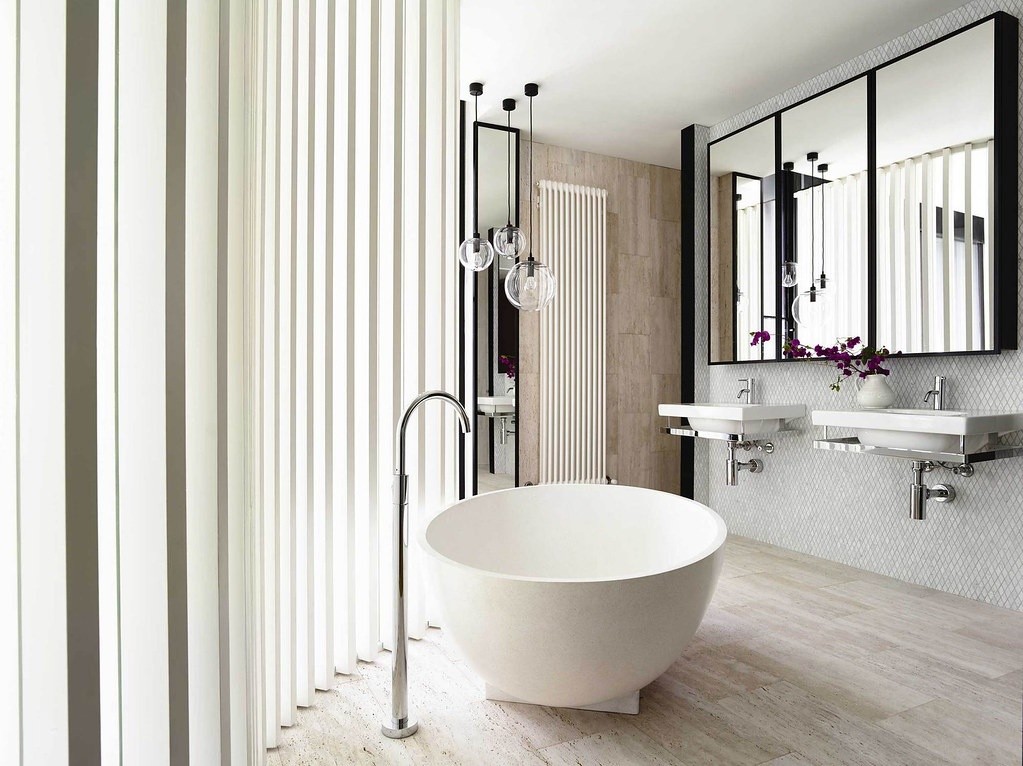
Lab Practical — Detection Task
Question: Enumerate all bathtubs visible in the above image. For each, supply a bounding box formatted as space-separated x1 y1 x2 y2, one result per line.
417 482 728 716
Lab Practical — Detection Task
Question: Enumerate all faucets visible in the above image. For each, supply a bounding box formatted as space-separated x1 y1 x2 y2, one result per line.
736 377 757 404
505 385 514 395
923 374 947 410
380 388 472 741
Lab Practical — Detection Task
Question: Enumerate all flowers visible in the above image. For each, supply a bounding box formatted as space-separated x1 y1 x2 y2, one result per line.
744 329 903 391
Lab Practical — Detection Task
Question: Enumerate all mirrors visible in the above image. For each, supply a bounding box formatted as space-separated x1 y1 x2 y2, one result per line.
471 121 519 494
731 171 764 362
779 69 871 362
873 10 1019 357
707 112 778 365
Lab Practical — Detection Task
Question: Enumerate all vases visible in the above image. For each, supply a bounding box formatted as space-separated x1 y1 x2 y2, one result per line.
855 375 897 411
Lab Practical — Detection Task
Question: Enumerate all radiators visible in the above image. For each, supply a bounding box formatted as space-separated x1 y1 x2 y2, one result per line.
535 180 608 484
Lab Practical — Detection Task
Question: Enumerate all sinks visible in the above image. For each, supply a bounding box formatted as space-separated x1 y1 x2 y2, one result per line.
476 394 515 413
810 406 1023 455
656 403 806 437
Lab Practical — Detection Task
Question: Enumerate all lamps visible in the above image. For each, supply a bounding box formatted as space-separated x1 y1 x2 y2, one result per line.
783 150 835 327
505 84 558 315
459 82 494 273
493 98 525 260
